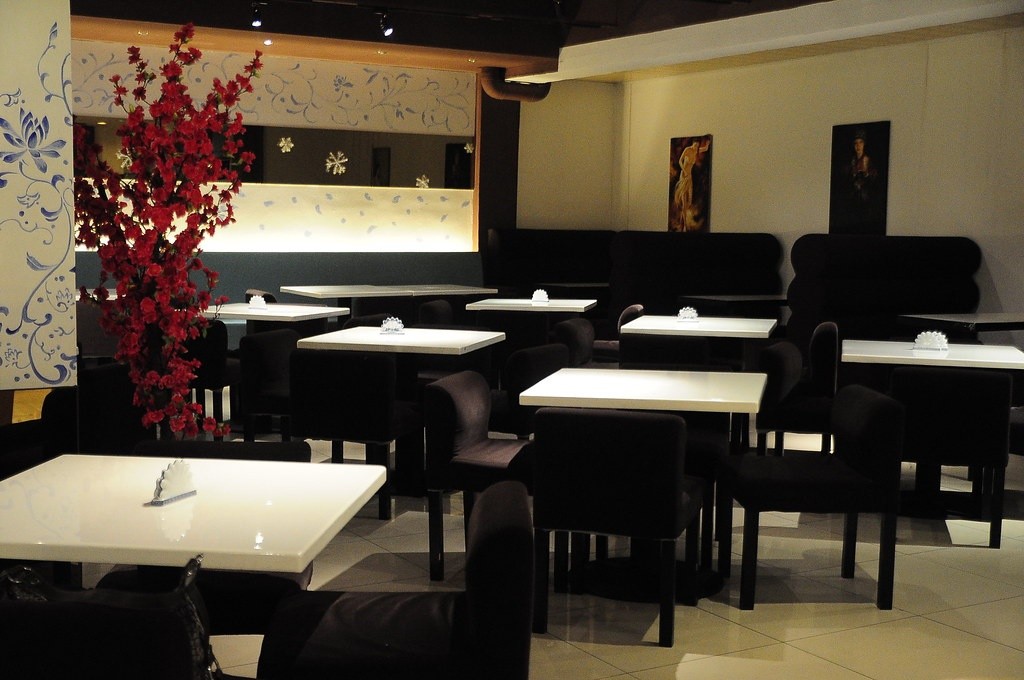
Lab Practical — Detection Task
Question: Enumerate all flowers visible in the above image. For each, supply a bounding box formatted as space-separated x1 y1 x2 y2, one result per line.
74 19 265 434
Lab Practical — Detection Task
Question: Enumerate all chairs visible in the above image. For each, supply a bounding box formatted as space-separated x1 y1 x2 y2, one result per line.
0 553 255 680
95 442 314 635
887 365 1013 549
716 385 907 610
258 480 540 680
422 370 535 581
532 407 703 648
0 271 839 472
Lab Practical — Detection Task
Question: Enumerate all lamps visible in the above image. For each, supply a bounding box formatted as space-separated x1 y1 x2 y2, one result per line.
249 2 270 27
377 14 393 36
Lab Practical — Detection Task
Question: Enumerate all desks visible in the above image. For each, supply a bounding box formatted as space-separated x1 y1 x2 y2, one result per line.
898 313 1024 332
536 282 611 288
519 367 768 413
0 453 388 574
465 298 598 313
678 293 789 307
379 285 499 296
280 285 412 323
620 315 777 339
296 325 507 355
841 338 1024 371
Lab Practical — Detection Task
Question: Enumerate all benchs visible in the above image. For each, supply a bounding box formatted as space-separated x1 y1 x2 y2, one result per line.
488 228 615 293
785 232 982 370
590 232 786 340
